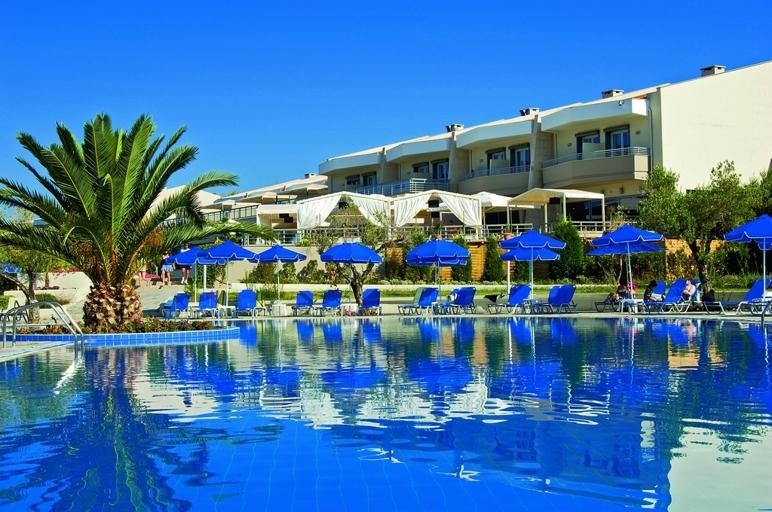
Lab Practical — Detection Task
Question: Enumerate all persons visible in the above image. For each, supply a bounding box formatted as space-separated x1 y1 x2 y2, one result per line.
159 248 176 287
677 277 696 304
178 242 192 285
643 280 663 302
607 278 638 303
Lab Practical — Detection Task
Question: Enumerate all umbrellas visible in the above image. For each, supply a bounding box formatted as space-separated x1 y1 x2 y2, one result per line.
256 243 305 301
591 224 663 299
499 230 567 300
164 246 225 304
724 215 772 298
405 237 469 300
320 241 382 304
193 239 258 306
587 241 664 298
502 247 560 299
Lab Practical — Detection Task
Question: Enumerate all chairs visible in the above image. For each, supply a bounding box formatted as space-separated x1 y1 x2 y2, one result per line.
398 282 578 316
644 320 692 347
508 319 579 349
594 276 770 317
354 288 383 316
296 318 382 347
313 287 344 317
237 320 258 347
418 318 475 345
159 289 267 317
291 290 317 316
